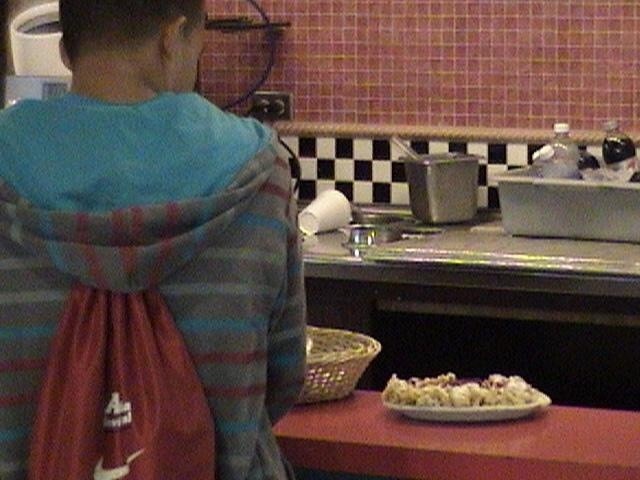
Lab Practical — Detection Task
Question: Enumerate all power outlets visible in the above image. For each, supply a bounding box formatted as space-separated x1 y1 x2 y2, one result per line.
249 93 295 122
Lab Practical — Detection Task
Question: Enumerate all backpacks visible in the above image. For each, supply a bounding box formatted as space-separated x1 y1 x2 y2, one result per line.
27 268 218 479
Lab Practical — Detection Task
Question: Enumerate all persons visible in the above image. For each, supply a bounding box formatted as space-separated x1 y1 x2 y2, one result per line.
0 1 308 480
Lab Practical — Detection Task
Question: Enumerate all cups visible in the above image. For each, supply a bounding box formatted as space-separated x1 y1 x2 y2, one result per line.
297 189 351 237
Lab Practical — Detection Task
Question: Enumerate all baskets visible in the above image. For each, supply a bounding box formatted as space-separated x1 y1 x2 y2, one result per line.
296 325 381 405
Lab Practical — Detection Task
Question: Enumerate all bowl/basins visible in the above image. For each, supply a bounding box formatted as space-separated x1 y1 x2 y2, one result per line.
400 154 480 225
494 166 639 242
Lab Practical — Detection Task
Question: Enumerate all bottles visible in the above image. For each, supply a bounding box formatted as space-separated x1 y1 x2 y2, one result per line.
577 145 601 181
528 150 546 178
539 144 584 180
602 120 640 183
547 123 579 170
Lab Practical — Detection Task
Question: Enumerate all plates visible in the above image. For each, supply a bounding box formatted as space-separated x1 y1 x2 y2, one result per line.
381 386 552 422
